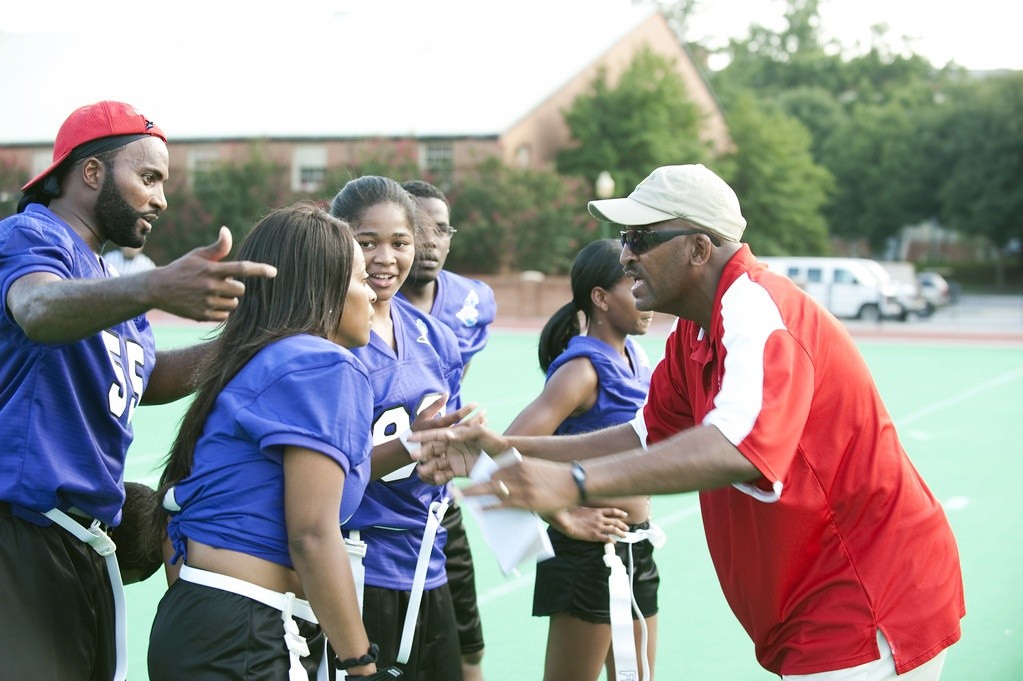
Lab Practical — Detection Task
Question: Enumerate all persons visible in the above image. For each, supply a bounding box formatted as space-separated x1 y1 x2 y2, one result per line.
0 101 280 681
500 238 661 681
399 181 496 681
105 481 164 586
327 174 485 680
146 200 378 681
407 164 968 681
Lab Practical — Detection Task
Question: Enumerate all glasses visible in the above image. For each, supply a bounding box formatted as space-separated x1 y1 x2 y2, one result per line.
413 226 458 238
618 229 722 256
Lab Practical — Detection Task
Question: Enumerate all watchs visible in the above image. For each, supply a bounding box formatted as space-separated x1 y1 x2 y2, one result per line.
570 460 590 506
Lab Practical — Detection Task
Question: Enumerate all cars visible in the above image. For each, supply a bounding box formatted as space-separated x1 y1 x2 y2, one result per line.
916 273 955 311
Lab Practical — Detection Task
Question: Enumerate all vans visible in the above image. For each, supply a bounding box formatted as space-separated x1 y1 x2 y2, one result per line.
755 252 891 325
870 258 924 320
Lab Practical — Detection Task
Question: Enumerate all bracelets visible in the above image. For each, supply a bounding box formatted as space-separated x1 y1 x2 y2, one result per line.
398 428 421 458
335 643 380 670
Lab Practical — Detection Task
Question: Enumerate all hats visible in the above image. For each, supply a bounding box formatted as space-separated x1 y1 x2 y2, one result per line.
587 162 748 243
21 101 167 193
